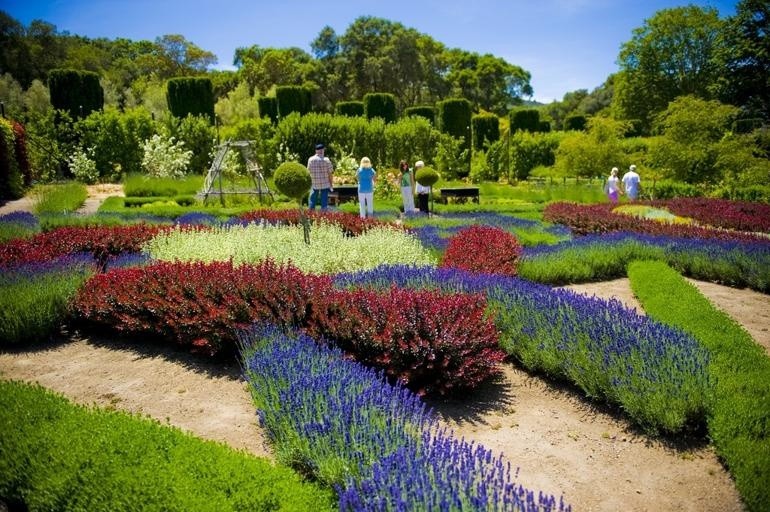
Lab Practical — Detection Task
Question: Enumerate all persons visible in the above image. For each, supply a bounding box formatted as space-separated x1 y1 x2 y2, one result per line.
397 160 414 215
605 166 623 205
414 161 432 216
355 157 377 219
621 164 641 202
306 143 334 211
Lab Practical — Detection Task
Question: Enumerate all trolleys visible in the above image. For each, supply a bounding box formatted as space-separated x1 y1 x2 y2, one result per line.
28 134 95 184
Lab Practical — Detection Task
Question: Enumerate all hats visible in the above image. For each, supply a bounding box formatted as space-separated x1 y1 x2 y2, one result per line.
316 144 324 150
